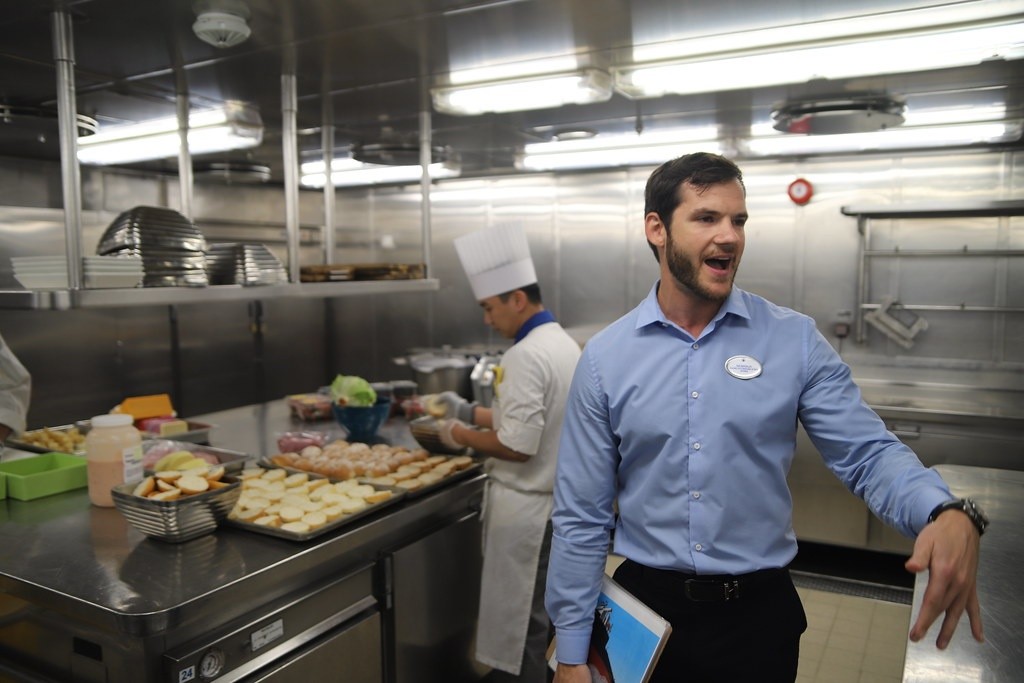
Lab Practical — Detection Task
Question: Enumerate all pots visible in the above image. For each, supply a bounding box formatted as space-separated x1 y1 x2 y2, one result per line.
408 354 470 402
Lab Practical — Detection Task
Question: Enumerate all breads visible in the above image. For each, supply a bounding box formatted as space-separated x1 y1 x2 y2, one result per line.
132 438 472 533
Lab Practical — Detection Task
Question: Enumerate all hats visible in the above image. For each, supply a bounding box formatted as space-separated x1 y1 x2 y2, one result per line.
453 221 537 301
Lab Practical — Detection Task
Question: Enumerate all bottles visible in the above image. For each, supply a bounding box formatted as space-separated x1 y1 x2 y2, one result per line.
87 413 144 507
470 351 500 408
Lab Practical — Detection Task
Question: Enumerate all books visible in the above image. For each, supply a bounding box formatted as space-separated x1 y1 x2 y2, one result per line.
546 569 672 683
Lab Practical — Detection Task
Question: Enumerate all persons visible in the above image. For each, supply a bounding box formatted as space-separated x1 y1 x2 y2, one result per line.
0 331 34 445
427 222 584 683
545 150 985 683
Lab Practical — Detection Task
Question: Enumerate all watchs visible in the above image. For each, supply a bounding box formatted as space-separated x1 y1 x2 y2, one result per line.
927 495 990 539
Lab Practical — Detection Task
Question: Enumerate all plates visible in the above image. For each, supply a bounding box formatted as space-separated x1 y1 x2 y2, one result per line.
300 264 424 282
10 205 290 290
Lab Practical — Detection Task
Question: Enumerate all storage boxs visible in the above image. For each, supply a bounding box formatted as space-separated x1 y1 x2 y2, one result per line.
0 451 88 501
285 393 333 422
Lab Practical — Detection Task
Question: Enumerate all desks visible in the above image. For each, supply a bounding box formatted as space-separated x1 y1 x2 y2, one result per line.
901 465 1024 683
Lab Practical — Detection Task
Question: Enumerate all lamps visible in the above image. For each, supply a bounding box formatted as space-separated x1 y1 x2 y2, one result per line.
77 118 266 166
429 66 612 117
606 13 1024 101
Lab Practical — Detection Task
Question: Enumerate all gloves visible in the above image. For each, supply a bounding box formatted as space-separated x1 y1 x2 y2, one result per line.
432 391 478 448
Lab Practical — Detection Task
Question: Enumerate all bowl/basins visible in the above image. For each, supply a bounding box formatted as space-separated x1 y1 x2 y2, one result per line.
332 397 392 443
411 416 468 455
277 432 326 454
110 474 243 543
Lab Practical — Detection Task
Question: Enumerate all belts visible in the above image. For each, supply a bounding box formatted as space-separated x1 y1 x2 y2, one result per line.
617 565 792 603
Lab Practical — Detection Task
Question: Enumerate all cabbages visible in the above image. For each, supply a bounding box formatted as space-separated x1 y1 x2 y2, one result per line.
330 375 376 406
